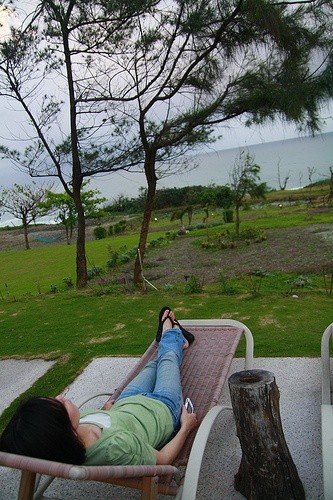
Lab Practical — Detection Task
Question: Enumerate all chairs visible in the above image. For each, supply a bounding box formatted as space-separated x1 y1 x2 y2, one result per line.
1 320 254 500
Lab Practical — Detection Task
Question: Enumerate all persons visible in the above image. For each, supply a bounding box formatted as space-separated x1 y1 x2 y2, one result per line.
0 307 201 472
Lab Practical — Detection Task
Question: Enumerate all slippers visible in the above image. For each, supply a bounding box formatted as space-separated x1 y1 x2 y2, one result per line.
173 319 194 344
155 306 174 343
